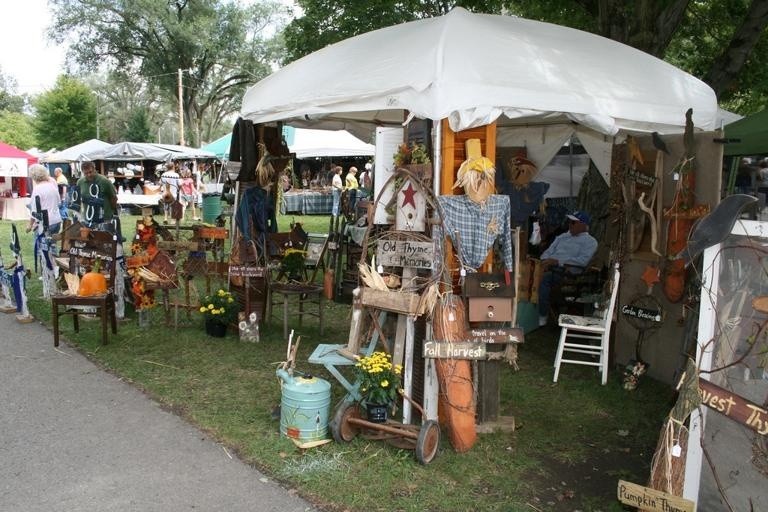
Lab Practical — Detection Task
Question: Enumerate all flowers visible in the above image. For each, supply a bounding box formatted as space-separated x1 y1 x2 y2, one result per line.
353 351 403 406
198 290 239 324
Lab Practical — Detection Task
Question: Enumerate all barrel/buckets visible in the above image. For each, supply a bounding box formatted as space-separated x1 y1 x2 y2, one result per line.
273 368 332 442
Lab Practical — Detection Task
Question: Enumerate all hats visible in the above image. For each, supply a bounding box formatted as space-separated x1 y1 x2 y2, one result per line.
565 210 591 225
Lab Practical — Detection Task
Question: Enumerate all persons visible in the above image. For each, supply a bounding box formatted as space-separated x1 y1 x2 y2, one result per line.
178 169 200 221
359 163 373 191
160 163 179 224
331 166 344 217
345 166 358 209
26 164 62 282
76 161 118 229
538 211 598 327
54 167 69 220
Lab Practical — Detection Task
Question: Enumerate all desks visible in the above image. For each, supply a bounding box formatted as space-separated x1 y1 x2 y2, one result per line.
116 195 162 205
281 189 367 215
0 197 31 221
203 183 225 196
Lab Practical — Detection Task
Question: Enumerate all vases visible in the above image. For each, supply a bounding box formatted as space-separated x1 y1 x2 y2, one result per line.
205 320 228 337
367 404 387 422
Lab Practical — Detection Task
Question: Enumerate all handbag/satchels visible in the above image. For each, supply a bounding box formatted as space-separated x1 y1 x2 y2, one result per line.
463 270 514 322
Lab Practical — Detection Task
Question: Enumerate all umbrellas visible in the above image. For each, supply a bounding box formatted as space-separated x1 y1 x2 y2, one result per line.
238 6 745 200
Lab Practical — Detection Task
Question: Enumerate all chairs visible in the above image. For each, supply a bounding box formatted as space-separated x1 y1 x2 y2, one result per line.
271 232 333 305
268 233 330 340
554 262 621 385
193 227 232 294
51 231 117 347
